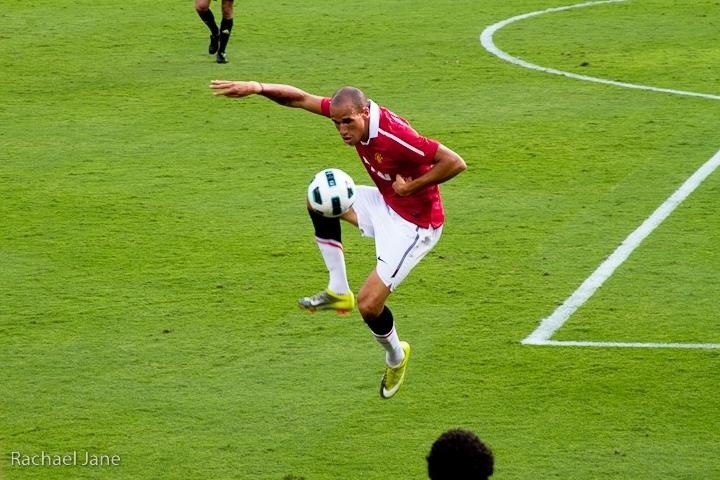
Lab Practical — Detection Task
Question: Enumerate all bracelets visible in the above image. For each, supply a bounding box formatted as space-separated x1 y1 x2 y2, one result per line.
259 81 265 95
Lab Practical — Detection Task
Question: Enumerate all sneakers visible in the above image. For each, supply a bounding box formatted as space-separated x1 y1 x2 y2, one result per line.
378 340 410 399
208 27 228 63
296 289 354 315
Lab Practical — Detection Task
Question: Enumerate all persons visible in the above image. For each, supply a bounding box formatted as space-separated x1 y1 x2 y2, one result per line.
423 430 496 479
209 77 468 401
194 1 236 64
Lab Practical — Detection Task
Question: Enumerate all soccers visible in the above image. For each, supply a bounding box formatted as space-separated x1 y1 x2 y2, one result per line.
308 168 355 218
